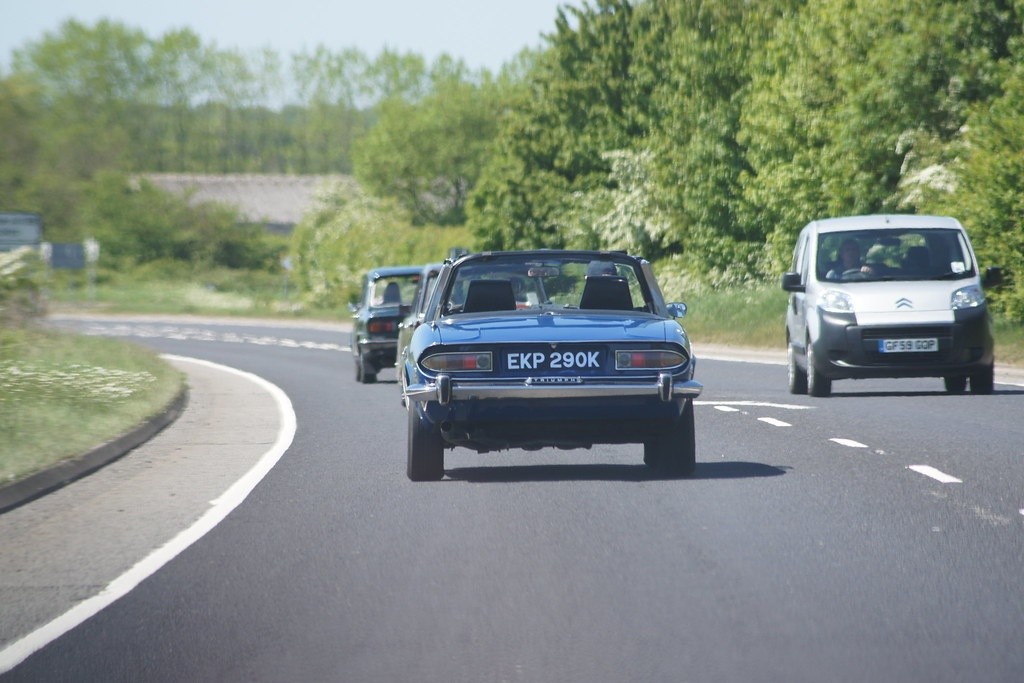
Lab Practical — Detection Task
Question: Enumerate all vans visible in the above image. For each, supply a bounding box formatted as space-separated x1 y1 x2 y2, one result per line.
779 211 1005 394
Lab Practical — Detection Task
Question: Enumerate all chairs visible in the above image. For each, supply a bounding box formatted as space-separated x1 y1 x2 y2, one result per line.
582 275 634 311
901 246 933 280
462 279 516 312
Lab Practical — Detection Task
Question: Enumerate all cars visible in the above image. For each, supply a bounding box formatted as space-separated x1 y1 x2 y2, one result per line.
399 248 699 481
344 265 426 386
392 260 442 407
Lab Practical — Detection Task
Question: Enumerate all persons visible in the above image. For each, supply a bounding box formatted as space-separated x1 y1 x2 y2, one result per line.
824 239 876 280
584 261 618 282
